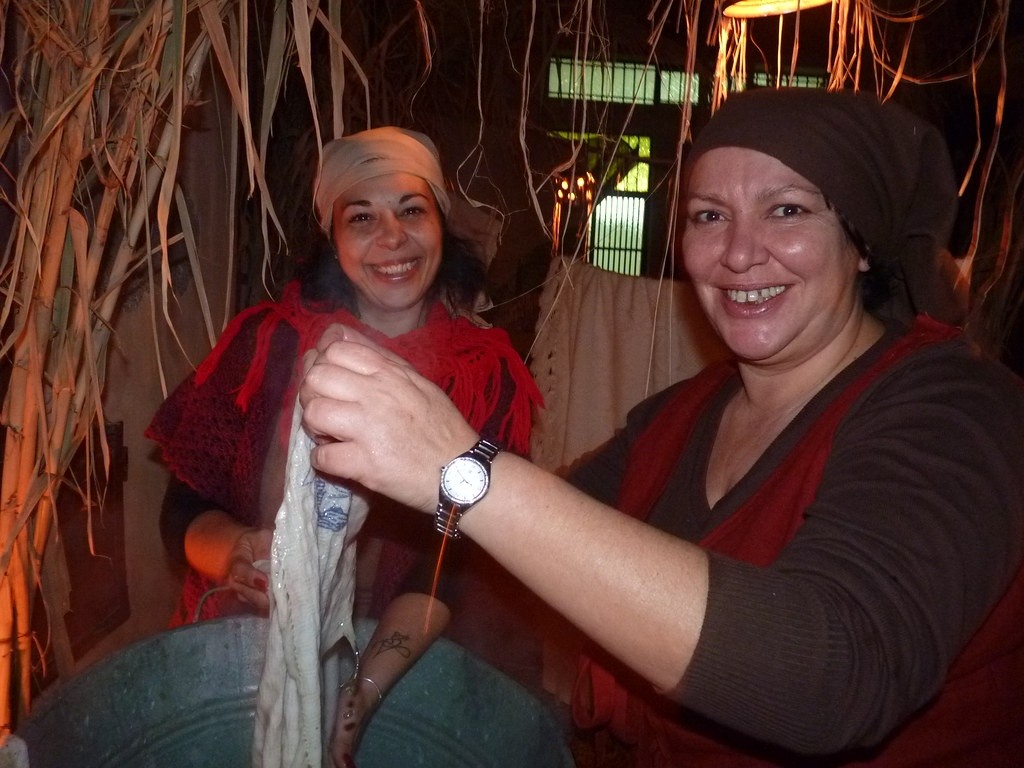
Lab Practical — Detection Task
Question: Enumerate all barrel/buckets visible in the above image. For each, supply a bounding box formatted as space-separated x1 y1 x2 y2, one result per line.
0 586 578 768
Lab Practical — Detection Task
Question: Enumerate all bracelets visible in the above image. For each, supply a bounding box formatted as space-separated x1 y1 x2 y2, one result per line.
350 677 384 702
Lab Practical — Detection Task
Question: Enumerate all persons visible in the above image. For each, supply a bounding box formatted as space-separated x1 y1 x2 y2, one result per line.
300 85 1024 768
145 125 547 768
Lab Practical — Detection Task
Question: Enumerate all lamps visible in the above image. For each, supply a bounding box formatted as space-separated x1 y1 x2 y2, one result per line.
721 0 835 20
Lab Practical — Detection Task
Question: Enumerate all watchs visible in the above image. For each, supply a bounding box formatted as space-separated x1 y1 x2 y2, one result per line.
434 437 504 543
